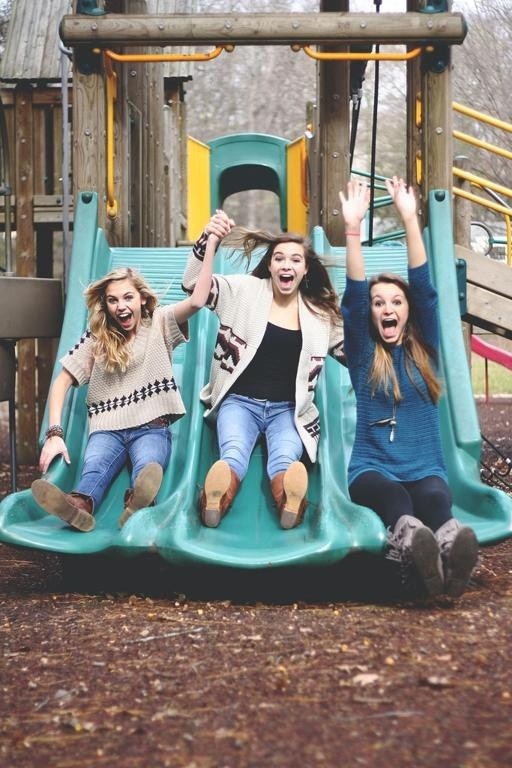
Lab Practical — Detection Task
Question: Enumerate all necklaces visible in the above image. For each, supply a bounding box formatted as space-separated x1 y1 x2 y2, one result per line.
368 343 404 442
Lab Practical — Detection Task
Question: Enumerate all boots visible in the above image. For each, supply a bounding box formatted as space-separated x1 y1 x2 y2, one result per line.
271 461 308 529
386 515 445 597
119 462 163 529
31 479 96 532
200 460 240 527
434 518 479 597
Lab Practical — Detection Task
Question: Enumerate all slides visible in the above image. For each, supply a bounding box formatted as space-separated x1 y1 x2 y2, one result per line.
0 188 511 569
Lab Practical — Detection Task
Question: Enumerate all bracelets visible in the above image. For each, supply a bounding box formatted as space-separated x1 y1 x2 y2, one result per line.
44 425 64 438
344 231 361 237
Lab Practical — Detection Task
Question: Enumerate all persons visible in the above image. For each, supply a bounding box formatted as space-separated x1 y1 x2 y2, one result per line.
29 209 233 533
181 210 345 529
337 175 480 598
